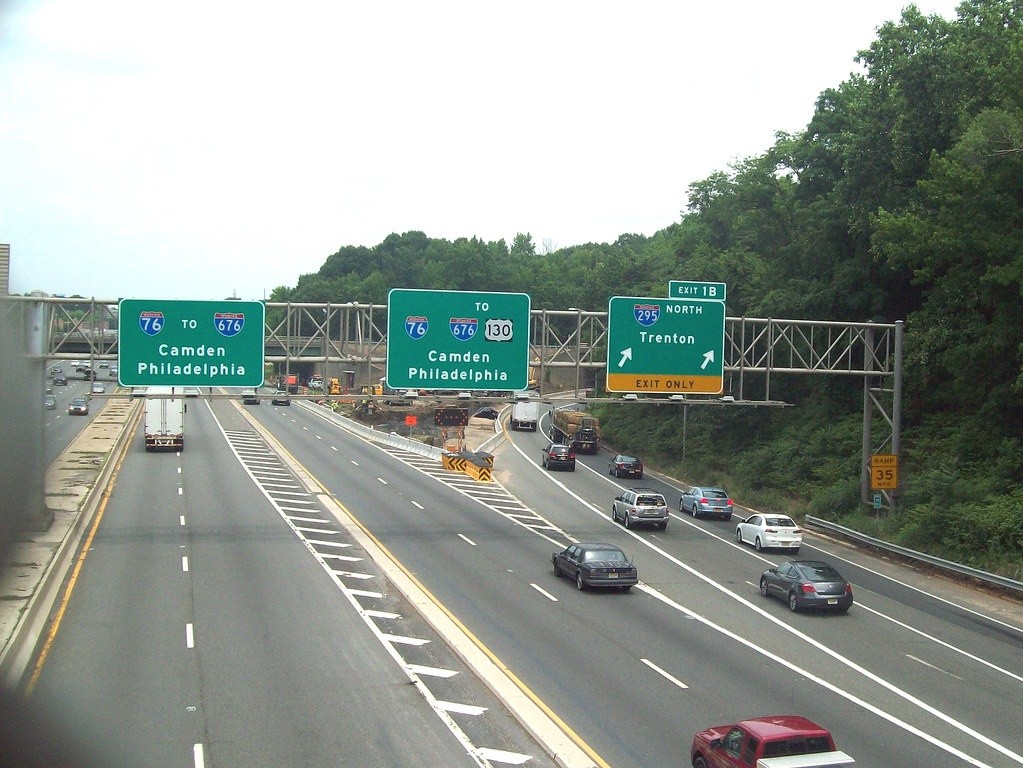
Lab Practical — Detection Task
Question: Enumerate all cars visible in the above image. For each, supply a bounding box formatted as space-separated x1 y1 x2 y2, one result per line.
271 390 292 406
760 559 854 613
735 513 803 555
67 397 90 416
49 360 118 377
45 382 54 394
89 382 107 394
608 454 644 480
45 395 57 410
379 376 542 402
550 542 639 594
244 394 261 405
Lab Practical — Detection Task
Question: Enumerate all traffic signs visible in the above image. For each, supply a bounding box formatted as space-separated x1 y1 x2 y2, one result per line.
608 295 727 395
385 288 531 392
117 297 265 387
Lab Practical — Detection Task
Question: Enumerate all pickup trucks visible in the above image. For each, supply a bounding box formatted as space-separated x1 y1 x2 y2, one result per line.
691 715 858 768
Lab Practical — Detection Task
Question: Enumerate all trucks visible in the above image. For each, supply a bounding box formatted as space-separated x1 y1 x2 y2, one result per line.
279 374 299 395
144 385 188 454
509 400 539 432
548 405 602 456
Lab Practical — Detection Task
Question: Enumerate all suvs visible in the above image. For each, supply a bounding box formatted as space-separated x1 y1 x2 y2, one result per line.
679 485 734 521
612 486 670 533
308 375 324 389
83 369 98 381
52 373 68 386
542 442 576 472
